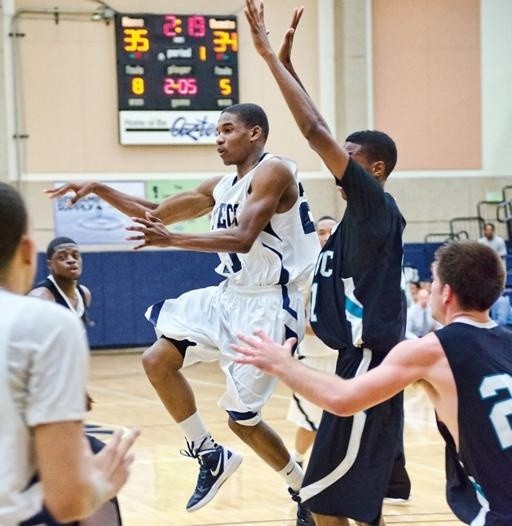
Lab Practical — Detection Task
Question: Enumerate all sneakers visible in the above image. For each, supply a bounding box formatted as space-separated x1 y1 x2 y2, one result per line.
186 444 243 513
288 487 316 526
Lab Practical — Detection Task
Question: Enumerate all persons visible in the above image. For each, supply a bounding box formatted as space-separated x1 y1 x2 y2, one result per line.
0 181 141 526
43 103 322 526
287 215 339 471
25 236 96 411
228 239 512 526
405 222 512 340
243 0 411 526
